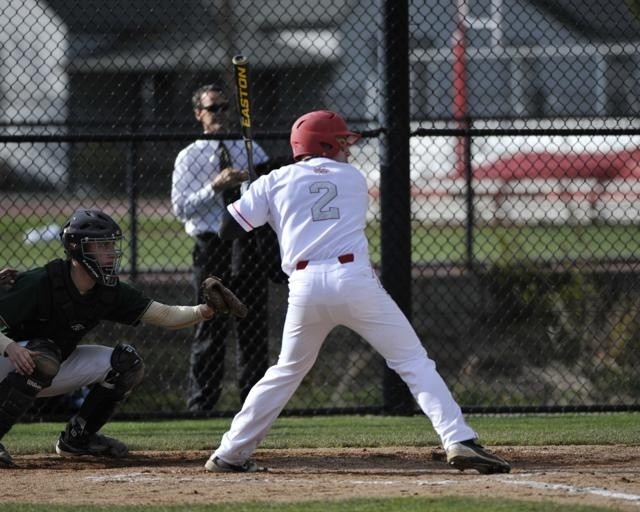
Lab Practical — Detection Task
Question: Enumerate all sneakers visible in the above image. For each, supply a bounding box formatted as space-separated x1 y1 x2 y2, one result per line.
0 444 14 467
204 454 258 472
56 433 129 461
446 444 511 473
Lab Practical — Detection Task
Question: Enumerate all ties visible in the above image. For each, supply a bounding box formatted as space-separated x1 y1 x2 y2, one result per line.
219 142 240 204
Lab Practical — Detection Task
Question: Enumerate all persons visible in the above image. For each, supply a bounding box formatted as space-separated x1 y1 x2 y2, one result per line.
204 109 510 475
171 84 269 418
0 209 247 469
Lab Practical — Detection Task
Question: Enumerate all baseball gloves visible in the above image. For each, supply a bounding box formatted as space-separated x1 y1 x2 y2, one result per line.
199 275 249 318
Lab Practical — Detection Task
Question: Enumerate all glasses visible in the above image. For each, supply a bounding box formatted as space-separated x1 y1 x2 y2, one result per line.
201 102 230 112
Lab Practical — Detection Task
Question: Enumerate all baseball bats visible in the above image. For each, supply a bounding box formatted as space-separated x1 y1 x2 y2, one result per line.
232 54 255 184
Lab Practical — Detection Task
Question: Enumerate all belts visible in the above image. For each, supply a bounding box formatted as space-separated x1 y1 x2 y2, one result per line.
296 254 353 270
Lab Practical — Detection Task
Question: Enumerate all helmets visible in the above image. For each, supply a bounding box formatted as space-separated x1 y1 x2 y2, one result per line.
291 109 362 161
59 208 125 289
